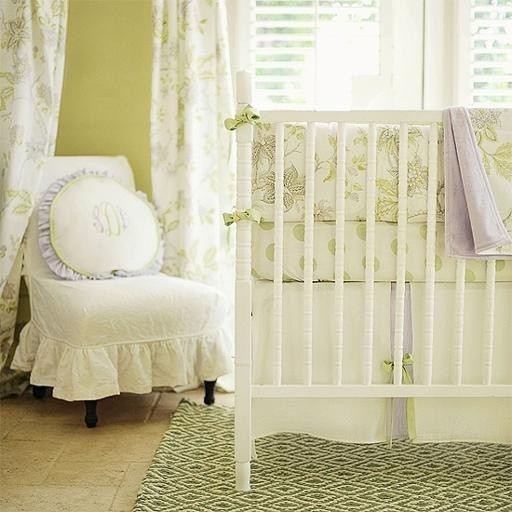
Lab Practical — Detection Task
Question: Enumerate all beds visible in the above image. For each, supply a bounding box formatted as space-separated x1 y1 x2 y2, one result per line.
229 72 511 494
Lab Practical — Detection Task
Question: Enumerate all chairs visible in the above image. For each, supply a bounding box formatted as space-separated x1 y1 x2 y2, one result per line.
17 151 227 428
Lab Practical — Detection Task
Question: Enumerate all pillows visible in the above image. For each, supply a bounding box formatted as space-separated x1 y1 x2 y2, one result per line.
252 107 508 224
38 167 163 279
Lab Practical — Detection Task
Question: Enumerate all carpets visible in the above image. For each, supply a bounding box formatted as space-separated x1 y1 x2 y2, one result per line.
116 399 510 511
